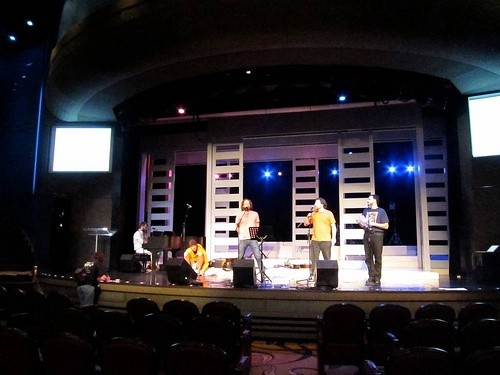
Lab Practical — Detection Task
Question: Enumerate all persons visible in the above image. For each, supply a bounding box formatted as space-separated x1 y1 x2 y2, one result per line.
304 198 336 281
358 195 389 284
184 239 209 275
75 252 104 307
235 199 261 272
133 222 160 269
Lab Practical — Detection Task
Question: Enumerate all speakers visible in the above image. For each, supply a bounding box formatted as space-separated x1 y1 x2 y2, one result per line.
314 259 339 290
165 258 197 286
233 258 256 289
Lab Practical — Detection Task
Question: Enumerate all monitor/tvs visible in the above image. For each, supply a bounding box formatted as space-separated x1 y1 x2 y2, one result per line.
48 123 116 175
467 89 500 158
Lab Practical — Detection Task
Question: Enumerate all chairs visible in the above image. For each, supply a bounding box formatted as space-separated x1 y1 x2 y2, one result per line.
315 300 500 375
0 286 253 375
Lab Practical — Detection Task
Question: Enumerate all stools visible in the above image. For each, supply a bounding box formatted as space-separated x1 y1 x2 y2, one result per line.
131 253 151 273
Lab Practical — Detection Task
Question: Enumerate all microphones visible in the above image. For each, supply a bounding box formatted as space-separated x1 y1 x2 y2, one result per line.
246 205 248 208
186 203 192 208
310 205 314 210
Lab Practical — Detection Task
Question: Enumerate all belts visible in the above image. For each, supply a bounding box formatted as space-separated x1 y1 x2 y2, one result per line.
365 230 383 234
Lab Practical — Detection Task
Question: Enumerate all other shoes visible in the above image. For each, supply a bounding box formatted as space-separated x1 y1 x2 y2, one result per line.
309 276 313 280
375 280 379 285
146 269 152 274
366 279 375 283
